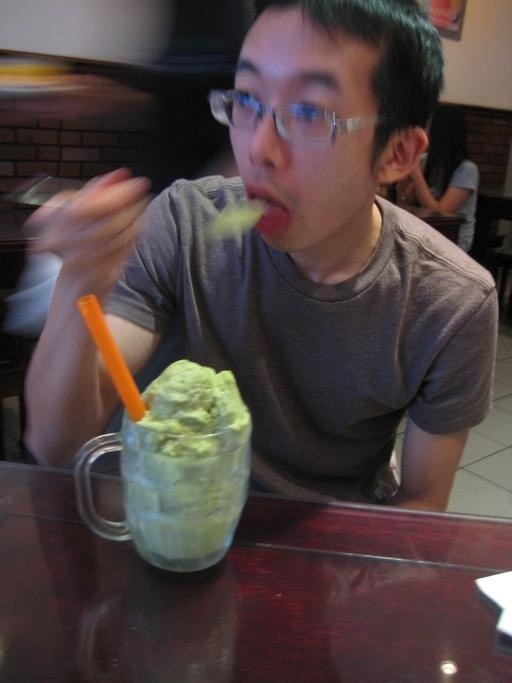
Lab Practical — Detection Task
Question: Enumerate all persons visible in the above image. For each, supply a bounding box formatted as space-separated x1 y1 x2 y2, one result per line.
398 104 482 254
17 0 501 518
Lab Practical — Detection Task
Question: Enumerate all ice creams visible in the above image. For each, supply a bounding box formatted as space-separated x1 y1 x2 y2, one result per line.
122 355 251 561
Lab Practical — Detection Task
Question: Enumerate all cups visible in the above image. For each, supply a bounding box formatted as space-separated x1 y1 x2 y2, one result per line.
73 400 252 573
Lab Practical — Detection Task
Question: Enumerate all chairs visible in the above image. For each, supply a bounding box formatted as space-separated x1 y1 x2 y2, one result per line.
469 193 512 319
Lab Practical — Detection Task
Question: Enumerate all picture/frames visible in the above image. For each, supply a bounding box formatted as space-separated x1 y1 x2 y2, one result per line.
419 0 466 41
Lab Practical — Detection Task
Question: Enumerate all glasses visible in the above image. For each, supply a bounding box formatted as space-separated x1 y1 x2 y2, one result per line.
202 86 382 148
